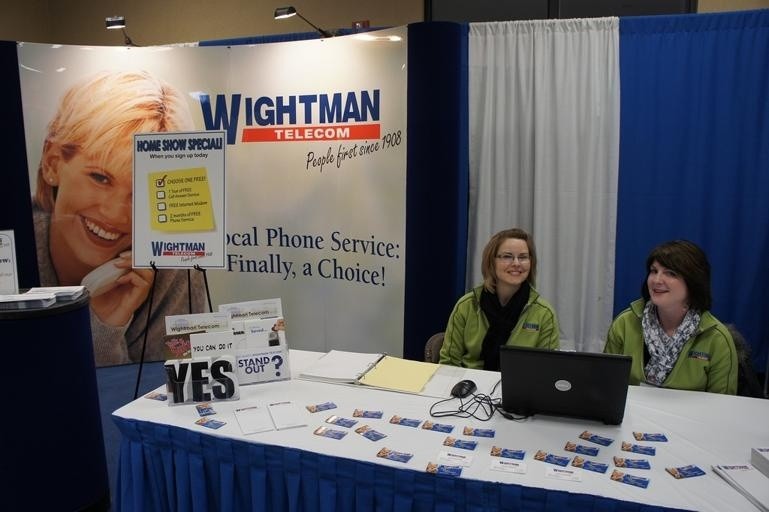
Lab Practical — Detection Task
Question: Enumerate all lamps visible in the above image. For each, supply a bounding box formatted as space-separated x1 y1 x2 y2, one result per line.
274 6 336 40
106 16 132 44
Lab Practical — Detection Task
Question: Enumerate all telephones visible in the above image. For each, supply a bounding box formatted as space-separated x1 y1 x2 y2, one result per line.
80 256 134 299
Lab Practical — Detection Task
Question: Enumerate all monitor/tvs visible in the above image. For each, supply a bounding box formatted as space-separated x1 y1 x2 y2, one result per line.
499 345 633 426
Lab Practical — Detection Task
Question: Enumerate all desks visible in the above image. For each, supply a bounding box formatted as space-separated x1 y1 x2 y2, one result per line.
109 349 769 512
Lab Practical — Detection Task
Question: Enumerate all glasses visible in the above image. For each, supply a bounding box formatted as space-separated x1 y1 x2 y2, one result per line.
495 254 531 264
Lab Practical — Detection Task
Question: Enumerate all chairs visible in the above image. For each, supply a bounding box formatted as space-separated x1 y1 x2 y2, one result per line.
425 333 444 363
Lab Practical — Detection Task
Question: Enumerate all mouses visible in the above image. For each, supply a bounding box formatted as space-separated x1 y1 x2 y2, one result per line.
451 380 478 399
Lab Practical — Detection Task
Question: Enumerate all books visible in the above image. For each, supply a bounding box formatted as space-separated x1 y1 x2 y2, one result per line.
300 343 442 395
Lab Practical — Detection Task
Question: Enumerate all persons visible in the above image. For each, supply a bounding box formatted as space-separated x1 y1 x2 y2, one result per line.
29 64 206 370
440 226 560 371
602 236 738 393
